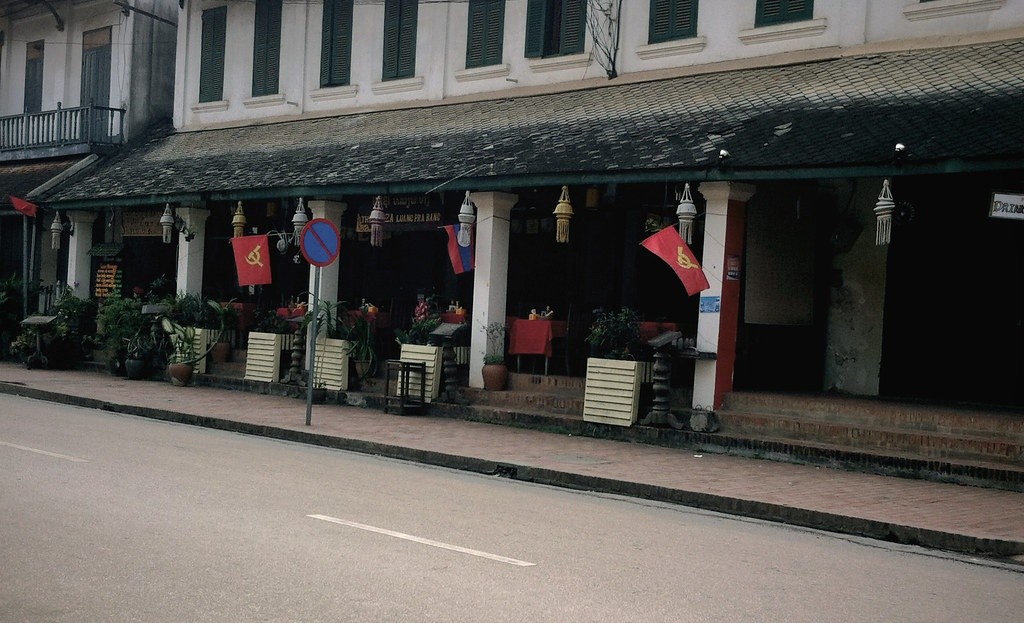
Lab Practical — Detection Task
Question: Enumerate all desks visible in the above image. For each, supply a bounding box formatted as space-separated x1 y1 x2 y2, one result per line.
346 311 392 329
633 321 697 340
504 317 585 376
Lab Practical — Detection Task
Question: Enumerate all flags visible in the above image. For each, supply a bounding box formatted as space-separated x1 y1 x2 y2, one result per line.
445 224 475 274
642 225 710 296
10 195 38 217
232 235 272 285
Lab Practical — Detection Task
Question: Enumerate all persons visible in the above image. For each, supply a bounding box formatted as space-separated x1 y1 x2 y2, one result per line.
133 288 148 304
159 271 176 301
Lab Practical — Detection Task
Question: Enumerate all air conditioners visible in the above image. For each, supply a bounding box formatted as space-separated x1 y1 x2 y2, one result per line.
482 324 512 390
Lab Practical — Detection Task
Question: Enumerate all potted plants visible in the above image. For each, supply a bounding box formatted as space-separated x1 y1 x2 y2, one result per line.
582 306 654 427
10 273 243 387
345 342 379 385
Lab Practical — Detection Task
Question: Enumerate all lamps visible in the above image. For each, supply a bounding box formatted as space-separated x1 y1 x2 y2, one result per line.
174 214 186 234
63 218 74 237
